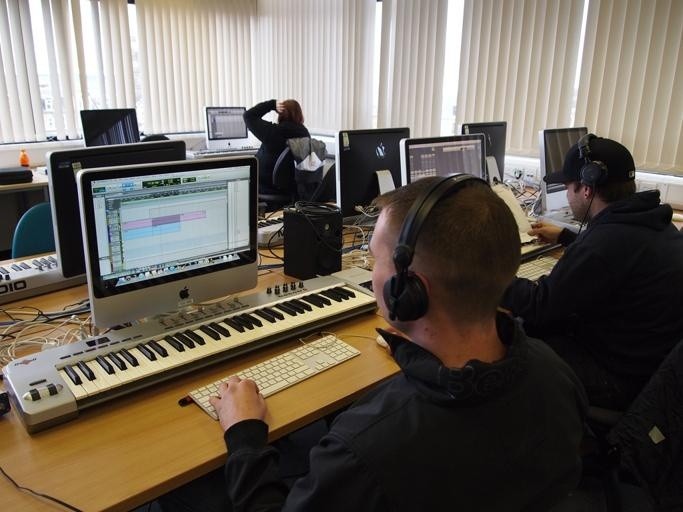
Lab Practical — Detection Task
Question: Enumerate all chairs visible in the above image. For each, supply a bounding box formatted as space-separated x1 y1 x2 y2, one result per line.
259 141 321 214
618 337 682 510
12 201 57 258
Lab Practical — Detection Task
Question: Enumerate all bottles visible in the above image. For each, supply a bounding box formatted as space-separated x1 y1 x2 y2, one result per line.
18 148 28 167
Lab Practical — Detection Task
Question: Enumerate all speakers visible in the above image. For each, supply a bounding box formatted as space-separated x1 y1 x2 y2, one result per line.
282 208 343 279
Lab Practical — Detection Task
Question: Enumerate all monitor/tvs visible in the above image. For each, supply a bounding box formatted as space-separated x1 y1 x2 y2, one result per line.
337 127 410 215
400 133 488 186
79 108 140 146
45 138 187 278
76 154 260 333
461 120 508 188
204 104 247 151
538 127 590 211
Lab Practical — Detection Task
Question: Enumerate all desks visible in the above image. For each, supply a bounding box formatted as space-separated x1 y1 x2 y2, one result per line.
1 185 683 511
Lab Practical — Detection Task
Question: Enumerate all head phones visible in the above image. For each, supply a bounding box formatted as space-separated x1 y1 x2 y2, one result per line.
383 174 488 320
576 135 608 194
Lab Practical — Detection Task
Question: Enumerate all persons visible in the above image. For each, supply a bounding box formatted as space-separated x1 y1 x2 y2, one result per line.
242 97 310 194
208 174 592 512
500 132 683 438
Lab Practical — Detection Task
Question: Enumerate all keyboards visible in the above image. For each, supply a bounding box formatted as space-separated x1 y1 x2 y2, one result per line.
186 334 360 420
516 256 557 281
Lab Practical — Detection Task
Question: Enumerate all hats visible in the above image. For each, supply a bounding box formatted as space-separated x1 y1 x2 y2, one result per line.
543 136 635 184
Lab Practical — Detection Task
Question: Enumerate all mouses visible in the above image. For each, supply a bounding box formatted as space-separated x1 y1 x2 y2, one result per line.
376 334 387 347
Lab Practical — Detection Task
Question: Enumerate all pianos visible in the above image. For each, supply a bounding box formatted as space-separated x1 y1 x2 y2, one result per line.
258 215 285 248
1 275 378 436
0 254 87 303
